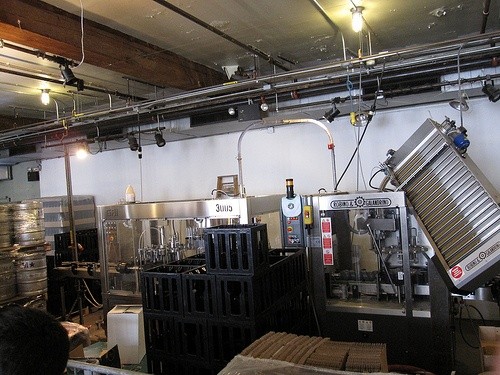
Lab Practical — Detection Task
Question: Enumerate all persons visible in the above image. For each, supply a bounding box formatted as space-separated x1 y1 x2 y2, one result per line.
0 302 69 375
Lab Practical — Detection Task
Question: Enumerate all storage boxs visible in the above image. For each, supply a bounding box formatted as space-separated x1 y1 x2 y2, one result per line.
107 225 305 375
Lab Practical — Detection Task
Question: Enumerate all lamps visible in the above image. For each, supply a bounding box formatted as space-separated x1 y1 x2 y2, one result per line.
350 6 364 32
323 97 344 123
449 92 470 112
154 127 166 147
129 132 138 151
41 89 49 106
59 64 85 90
482 75 500 102
350 99 369 127
